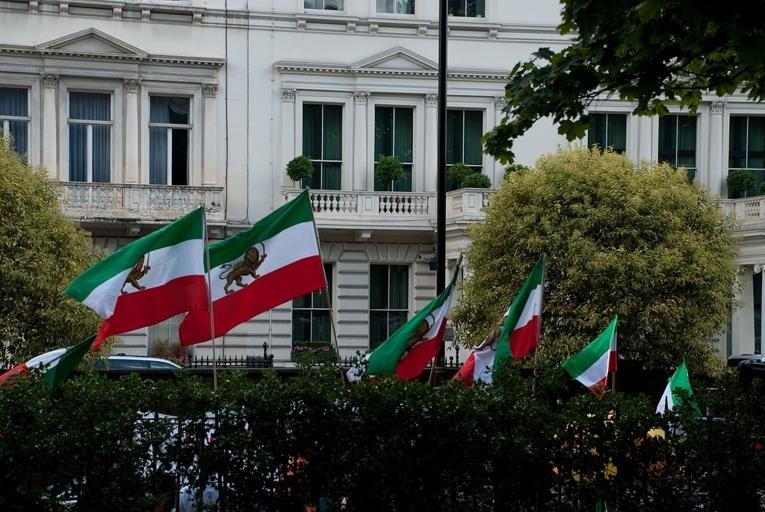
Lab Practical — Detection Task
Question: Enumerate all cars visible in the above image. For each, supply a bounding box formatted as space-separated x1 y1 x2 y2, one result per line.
727 352 765 387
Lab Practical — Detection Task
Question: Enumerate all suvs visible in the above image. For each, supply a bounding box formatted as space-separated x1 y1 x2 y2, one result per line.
93 355 245 457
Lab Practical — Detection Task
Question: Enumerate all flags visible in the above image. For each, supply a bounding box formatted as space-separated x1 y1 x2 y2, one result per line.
179 190 326 347
346 253 463 382
450 252 545 385
561 314 618 401
655 360 704 419
0 334 96 390
62 206 211 352
